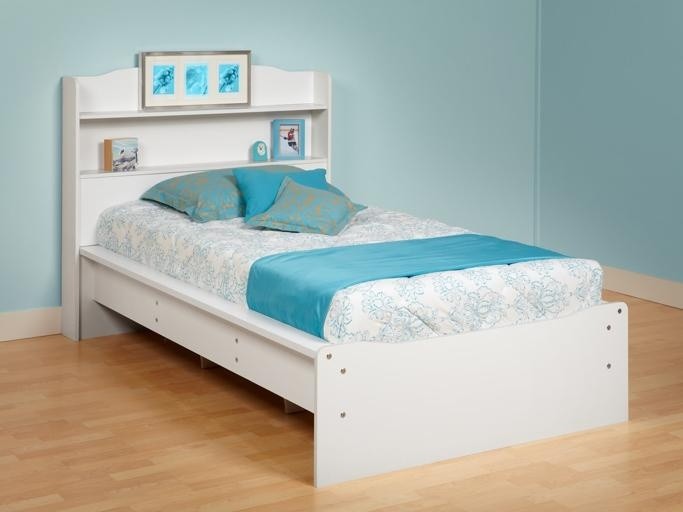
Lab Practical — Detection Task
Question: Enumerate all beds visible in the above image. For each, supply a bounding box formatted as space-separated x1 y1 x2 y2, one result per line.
61 49 630 488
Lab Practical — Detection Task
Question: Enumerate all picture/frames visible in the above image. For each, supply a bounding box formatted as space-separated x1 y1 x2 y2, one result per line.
273 119 305 161
138 51 251 112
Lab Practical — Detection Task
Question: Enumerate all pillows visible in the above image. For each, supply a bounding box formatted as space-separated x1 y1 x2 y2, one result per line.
245 176 369 235
138 163 349 223
231 167 328 224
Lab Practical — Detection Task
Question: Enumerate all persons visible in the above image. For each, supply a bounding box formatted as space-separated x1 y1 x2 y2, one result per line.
284 128 298 151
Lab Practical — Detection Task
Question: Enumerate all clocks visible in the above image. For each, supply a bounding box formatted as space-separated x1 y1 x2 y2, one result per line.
253 141 267 161
